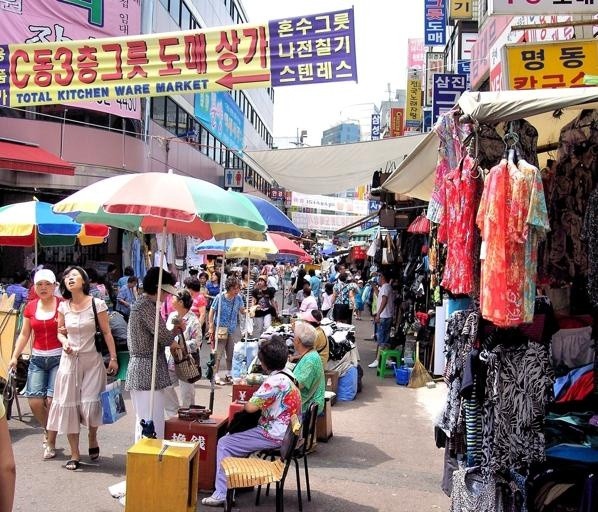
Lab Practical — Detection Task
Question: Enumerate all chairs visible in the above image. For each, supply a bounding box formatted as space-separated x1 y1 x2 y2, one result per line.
224 410 311 512
266 404 321 512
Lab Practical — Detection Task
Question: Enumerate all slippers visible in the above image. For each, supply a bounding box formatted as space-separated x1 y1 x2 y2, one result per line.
88 447 99 461
65 459 79 470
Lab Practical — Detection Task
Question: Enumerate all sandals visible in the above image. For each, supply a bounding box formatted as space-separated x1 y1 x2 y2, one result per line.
43 433 56 459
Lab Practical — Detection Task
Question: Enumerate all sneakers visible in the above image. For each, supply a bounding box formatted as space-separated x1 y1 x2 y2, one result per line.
201 496 235 506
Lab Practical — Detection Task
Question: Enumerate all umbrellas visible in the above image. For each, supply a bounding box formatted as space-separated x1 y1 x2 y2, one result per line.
207 185 300 352
0 194 109 273
195 231 314 337
53 168 301 438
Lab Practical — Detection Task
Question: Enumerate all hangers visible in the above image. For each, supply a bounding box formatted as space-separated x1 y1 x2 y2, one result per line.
466 467 485 484
500 130 524 164
453 137 487 179
500 328 529 354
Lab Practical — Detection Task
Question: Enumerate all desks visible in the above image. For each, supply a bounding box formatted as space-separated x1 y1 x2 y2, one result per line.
125 373 262 512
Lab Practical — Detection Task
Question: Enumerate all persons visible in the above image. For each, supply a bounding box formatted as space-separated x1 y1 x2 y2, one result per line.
15 268 62 459
105 255 395 506
46 262 117 471
0 397 15 512
7 272 28 310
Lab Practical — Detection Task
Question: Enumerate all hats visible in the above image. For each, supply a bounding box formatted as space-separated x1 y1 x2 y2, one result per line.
302 309 320 323
34 269 56 286
157 283 179 297
258 275 267 281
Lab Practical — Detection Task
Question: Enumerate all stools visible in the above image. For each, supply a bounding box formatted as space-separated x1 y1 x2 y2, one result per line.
375 349 401 380
315 390 334 444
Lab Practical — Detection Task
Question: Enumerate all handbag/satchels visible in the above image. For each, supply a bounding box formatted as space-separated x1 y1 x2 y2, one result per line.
100 385 127 425
228 410 262 432
95 330 109 351
10 354 30 394
174 352 202 384
218 327 228 340
